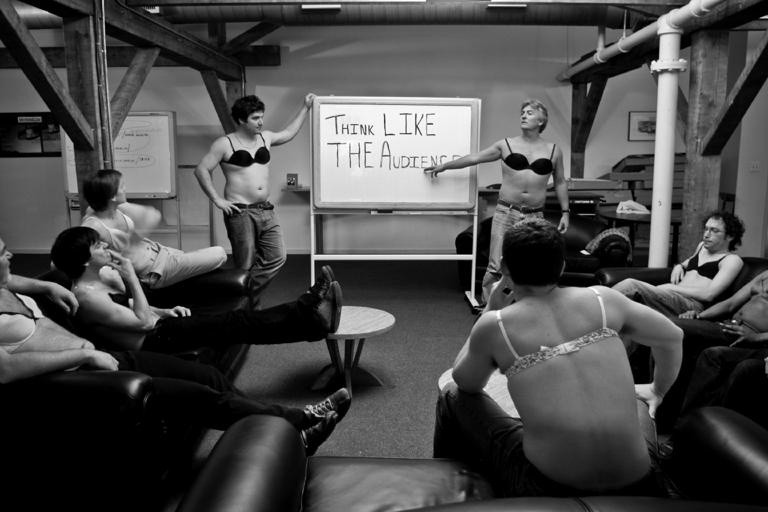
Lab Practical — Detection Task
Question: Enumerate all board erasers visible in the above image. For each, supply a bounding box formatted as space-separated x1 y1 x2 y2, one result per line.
377 210 392 213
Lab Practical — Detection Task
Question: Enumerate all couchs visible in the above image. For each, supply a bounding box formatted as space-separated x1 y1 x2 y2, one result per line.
1 246 256 512
590 251 768 378
648 319 767 410
176 405 767 512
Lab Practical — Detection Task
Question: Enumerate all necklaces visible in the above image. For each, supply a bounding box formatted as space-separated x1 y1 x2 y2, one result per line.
234 134 259 148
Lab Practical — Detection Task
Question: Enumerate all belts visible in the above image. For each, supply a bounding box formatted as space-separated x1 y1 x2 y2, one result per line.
497 199 545 214
236 201 274 210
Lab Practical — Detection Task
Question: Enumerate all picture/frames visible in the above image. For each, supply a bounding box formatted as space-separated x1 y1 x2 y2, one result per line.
626 109 661 142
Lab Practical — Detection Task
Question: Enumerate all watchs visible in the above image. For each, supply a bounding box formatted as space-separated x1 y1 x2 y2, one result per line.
561 209 571 213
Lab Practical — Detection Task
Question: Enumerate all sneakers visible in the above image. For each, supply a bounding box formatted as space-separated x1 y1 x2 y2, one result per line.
317 281 342 334
301 411 338 456
309 264 335 299
305 388 353 426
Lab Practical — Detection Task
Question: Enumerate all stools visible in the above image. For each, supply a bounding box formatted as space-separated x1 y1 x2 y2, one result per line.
310 304 397 400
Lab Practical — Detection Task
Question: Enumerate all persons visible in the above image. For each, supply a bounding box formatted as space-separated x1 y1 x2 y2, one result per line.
49 170 228 290
0 238 352 460
51 227 342 358
194 93 315 311
423 100 571 311
433 218 686 499
608 211 745 360
648 270 768 445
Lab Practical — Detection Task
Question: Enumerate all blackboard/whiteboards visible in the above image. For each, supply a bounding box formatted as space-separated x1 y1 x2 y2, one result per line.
313 96 478 210
60 111 177 200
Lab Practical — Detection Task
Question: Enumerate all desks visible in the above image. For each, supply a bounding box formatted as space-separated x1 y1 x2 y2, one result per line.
280 178 695 261
